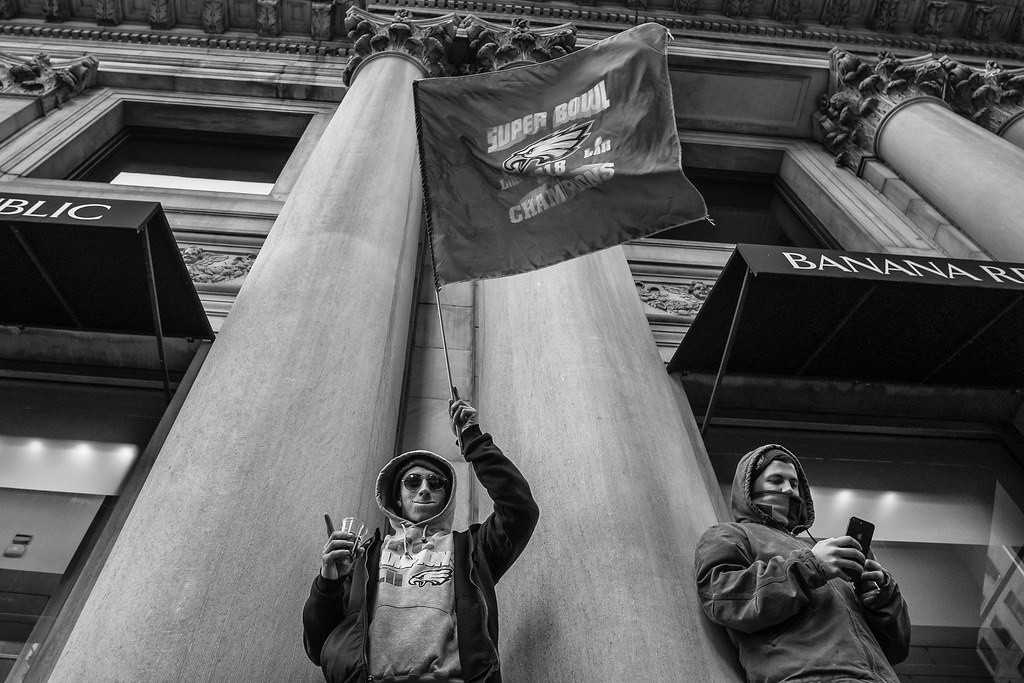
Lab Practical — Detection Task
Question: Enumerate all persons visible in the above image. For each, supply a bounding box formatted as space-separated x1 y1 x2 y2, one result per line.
302 386 540 683
694 444 911 683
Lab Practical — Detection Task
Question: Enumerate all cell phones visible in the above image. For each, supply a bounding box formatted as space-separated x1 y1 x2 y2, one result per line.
837 517 875 582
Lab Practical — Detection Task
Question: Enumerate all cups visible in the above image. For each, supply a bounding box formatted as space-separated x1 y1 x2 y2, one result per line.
850 575 880 604
336 516 368 563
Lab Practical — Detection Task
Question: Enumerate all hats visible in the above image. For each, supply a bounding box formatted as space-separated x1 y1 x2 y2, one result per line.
391 461 450 497
754 450 800 479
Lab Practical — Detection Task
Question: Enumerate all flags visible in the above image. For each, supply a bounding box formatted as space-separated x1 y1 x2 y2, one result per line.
413 21 710 289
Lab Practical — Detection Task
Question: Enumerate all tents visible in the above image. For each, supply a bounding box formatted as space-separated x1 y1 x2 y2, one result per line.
666 240 1024 439
0 192 216 403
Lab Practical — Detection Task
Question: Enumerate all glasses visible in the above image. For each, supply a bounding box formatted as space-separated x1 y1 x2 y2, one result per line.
398 473 448 492
